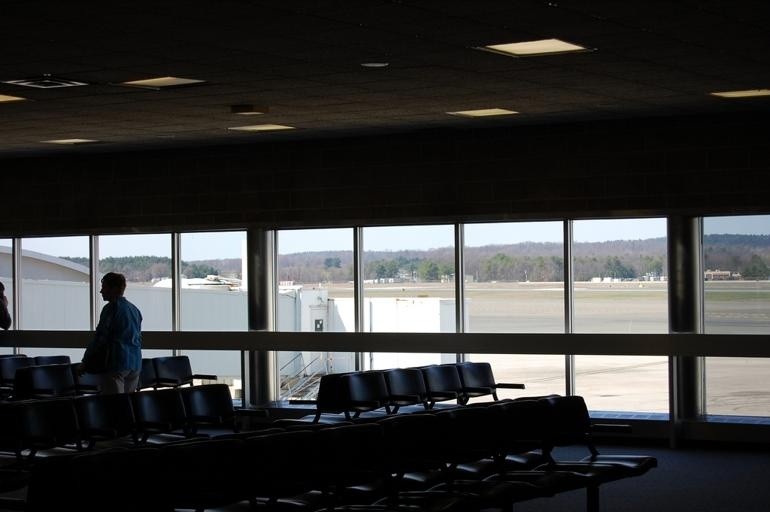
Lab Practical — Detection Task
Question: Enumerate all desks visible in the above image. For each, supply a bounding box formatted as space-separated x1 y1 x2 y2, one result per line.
237 400 317 417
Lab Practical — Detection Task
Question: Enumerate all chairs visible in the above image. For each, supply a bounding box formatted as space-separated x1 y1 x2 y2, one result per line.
233 394 659 511
1 354 268 510
289 362 525 424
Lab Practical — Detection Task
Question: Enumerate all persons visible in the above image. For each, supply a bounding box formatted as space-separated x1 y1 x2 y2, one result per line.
0 283 12 330
79 271 143 443
